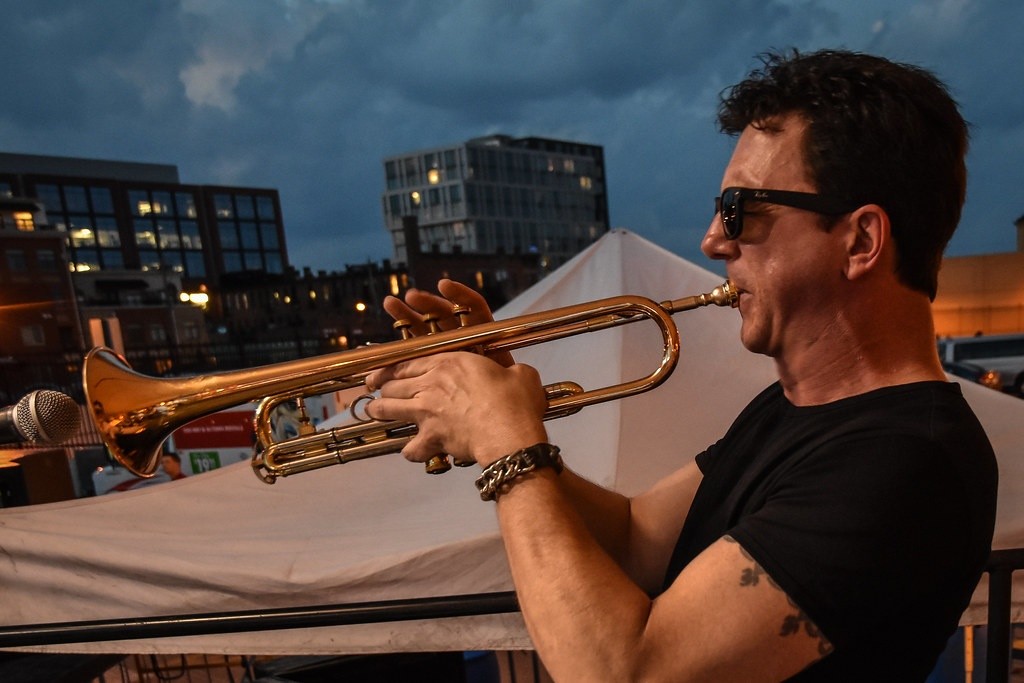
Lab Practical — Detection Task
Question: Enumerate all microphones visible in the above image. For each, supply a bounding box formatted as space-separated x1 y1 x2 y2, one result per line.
1 390 82 447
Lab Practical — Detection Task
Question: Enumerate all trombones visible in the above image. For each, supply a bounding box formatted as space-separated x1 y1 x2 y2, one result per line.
87 277 735 487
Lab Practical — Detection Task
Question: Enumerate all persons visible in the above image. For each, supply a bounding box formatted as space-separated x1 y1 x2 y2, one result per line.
364 52 998 683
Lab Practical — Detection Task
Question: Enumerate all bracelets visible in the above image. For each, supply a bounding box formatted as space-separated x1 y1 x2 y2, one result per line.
473 441 562 502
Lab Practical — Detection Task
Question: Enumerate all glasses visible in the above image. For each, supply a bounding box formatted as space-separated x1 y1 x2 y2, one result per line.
714 185 856 240
